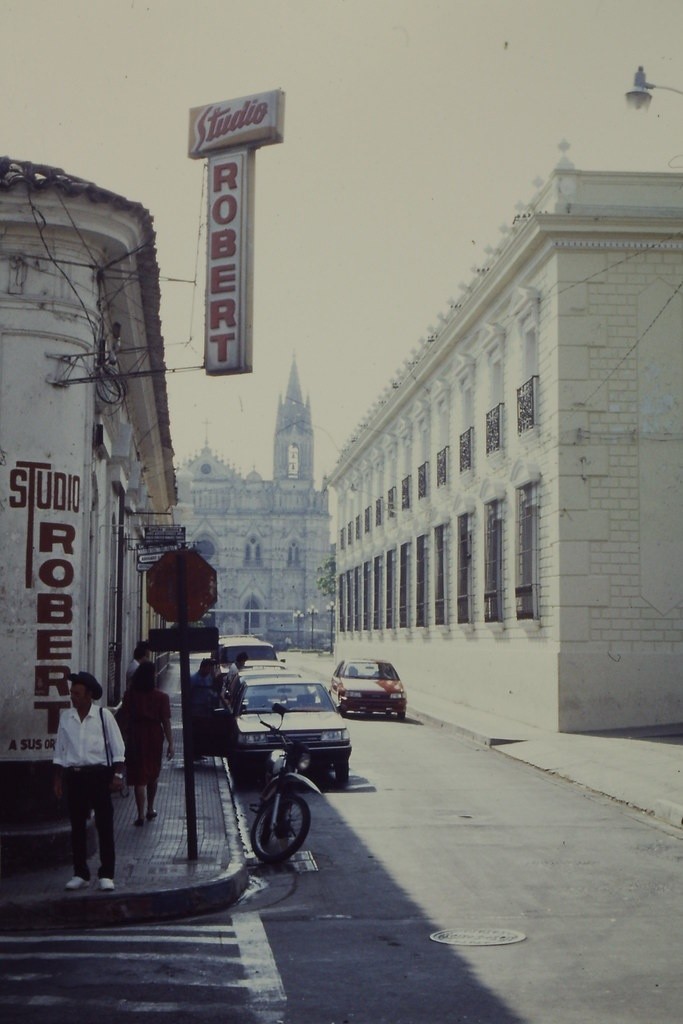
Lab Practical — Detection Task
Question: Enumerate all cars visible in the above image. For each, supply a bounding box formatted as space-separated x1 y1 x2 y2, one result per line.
329 658 407 720
217 635 353 789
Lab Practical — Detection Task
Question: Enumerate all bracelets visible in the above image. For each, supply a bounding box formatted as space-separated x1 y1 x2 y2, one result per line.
114 773 123 779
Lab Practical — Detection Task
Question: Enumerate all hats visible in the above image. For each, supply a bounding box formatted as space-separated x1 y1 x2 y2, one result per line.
68 672 102 700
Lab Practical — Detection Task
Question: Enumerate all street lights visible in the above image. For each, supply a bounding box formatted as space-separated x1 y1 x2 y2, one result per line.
306 605 318 650
294 610 305 649
326 600 336 654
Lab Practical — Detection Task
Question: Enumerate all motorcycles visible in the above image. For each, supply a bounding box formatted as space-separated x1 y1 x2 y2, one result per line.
248 702 323 864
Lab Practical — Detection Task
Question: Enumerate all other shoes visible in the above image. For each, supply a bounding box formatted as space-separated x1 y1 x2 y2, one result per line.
147 809 157 820
134 819 144 826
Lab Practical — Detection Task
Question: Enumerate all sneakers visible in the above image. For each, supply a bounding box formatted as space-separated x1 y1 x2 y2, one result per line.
66 877 90 889
99 878 114 890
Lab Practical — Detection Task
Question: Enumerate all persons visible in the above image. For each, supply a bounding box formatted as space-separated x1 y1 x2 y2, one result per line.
188 652 248 761
372 664 391 679
119 662 175 825
126 644 150 689
53 671 126 889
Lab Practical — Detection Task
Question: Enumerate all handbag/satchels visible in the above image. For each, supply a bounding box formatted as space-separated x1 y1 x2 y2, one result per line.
106 754 134 792
